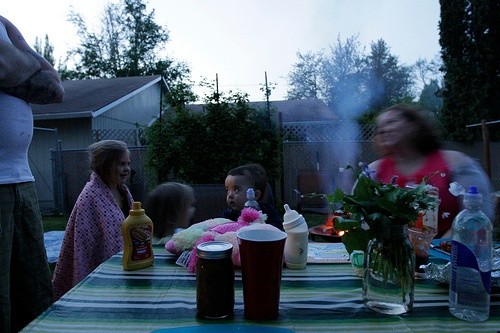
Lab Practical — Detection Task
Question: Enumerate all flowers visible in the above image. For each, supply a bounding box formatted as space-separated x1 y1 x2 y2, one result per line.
297 161 452 251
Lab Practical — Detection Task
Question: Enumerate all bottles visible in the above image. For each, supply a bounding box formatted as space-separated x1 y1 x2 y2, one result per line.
122 202 154 270
283 204 309 269
196 241 234 321
244 188 260 211
448 182 493 322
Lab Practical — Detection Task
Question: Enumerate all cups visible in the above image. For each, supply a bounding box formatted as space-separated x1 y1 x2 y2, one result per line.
236 230 287 321
408 228 438 276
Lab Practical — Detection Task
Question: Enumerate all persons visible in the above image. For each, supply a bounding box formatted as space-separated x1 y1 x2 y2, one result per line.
52 139 136 298
146 182 197 234
351 102 496 249
220 162 284 230
0 15 66 331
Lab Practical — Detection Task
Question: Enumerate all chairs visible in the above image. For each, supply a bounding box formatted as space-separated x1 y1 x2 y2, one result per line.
178 184 275 224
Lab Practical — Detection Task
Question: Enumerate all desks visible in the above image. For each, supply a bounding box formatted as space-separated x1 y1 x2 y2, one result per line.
18 239 500 333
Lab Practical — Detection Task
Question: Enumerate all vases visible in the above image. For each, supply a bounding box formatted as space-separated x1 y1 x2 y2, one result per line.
362 226 419 315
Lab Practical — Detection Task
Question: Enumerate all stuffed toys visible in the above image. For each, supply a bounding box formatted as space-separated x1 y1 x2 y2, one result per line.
166 207 266 269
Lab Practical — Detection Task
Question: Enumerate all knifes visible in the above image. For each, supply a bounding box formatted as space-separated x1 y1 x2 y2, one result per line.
430 243 451 256
